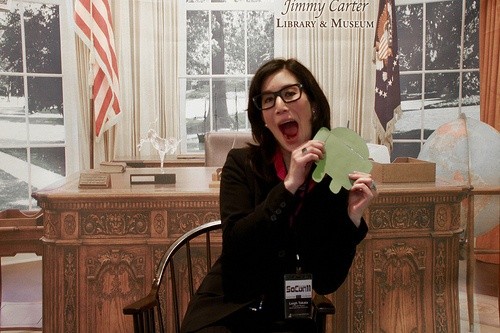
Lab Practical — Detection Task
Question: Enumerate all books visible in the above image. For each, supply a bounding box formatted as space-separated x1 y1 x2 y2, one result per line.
100 161 127 173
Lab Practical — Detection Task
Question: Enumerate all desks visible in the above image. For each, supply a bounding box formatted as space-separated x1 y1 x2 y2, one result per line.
33 166 473 333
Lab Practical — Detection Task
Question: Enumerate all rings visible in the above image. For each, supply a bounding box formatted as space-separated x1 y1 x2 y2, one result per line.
369 181 376 189
302 148 307 154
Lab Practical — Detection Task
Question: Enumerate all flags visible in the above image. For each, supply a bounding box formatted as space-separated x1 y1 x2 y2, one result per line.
73 0 123 143
372 0 403 157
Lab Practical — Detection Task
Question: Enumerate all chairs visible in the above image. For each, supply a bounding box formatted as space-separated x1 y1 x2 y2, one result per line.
204 134 253 166
123 219 336 333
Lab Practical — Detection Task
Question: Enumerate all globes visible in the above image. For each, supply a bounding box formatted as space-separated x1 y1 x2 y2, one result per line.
417 113 500 260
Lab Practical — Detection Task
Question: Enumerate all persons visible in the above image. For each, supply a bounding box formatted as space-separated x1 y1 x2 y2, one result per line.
180 60 383 333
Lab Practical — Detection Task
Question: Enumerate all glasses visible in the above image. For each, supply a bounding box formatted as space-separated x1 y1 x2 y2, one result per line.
251 83 304 111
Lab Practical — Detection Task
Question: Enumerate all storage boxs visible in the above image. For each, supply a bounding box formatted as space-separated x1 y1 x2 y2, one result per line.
371 158 436 184
